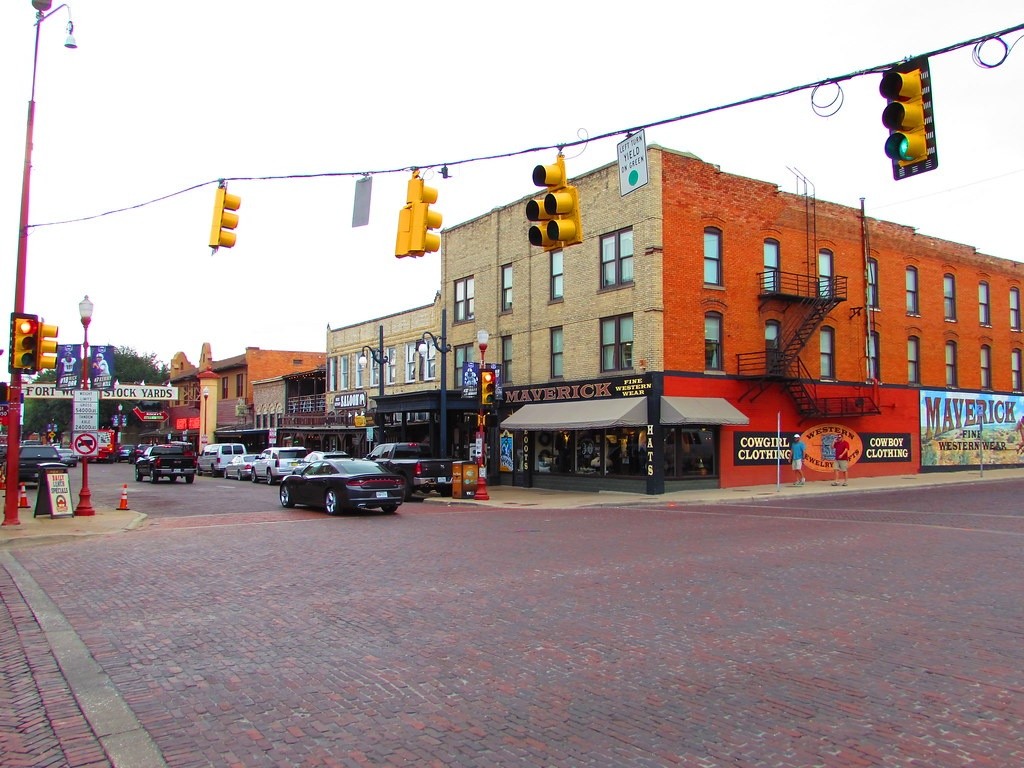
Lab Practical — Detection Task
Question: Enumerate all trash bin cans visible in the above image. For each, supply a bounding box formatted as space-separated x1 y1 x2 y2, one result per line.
453 461 479 499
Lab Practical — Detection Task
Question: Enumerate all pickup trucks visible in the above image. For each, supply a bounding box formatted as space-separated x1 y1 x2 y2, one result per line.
135 447 197 484
363 442 459 498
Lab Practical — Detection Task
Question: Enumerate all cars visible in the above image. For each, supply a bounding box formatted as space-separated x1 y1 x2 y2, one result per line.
279 458 406 517
18 446 62 489
20 440 43 445
251 446 308 485
128 443 157 464
114 445 135 463
56 449 78 467
222 454 262 481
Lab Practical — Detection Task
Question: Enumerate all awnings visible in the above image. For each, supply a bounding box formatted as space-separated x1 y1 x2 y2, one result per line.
500 396 648 429
139 427 199 435
659 397 750 425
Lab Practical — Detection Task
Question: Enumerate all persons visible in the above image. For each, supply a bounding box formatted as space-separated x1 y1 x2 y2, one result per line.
57 351 77 378
92 352 112 379
787 433 806 487
1014 416 1024 456
495 369 502 388
463 367 479 389
831 433 851 486
501 438 511 459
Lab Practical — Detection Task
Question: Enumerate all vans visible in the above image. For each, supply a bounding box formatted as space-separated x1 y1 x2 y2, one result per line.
196 443 247 478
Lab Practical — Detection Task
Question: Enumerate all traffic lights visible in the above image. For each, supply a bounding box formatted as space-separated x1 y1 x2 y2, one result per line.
8 312 38 375
476 368 496 408
208 187 241 249
394 179 442 258
879 55 939 180
34 320 59 371
525 158 583 252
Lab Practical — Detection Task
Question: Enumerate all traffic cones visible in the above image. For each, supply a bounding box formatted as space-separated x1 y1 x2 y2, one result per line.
17 483 32 508
115 484 131 510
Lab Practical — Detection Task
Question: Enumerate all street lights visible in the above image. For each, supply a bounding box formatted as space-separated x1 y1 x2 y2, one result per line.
71 294 96 516
418 332 447 454
203 386 209 435
475 330 490 500
1 3 78 525
358 346 384 445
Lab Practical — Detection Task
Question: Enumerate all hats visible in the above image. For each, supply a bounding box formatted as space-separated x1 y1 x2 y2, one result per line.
838 434 843 438
793 434 800 438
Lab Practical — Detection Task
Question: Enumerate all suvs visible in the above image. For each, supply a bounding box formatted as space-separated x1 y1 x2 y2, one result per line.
291 451 349 475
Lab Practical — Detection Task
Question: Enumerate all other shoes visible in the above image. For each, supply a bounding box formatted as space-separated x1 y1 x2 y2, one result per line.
793 481 800 486
842 483 848 486
832 482 838 486
797 482 805 486
